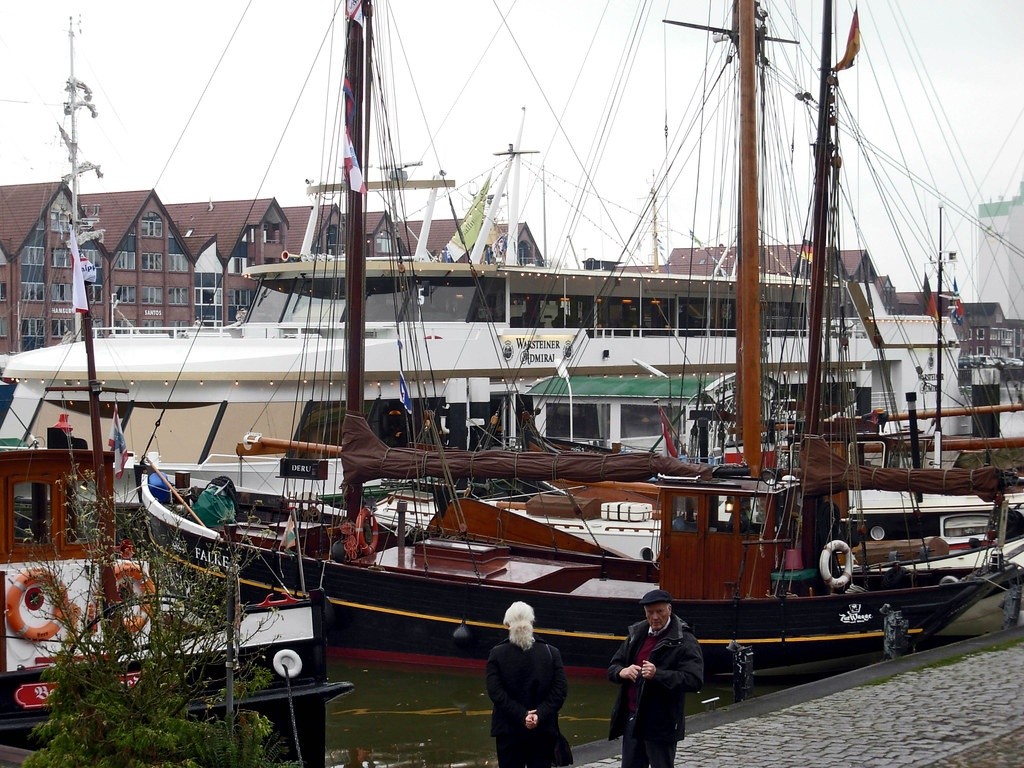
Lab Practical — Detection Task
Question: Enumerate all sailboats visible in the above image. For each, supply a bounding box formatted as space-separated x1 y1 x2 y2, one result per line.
0 0 1024 765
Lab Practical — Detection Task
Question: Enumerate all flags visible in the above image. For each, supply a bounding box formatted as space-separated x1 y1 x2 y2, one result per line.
801 240 813 261
68 226 96 310
951 277 964 325
342 0 366 193
343 74 356 143
831 6 862 72
109 403 128 479
924 271 939 320
661 411 677 458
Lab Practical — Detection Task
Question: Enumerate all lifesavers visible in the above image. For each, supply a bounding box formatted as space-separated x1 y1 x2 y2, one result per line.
355 507 379 556
820 540 854 588
113 563 155 633
8 567 69 641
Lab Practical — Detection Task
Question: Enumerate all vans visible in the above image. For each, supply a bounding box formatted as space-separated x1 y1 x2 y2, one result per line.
981 354 1007 367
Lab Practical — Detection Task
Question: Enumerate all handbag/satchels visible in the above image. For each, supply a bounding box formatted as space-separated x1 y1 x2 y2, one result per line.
552 730 573 768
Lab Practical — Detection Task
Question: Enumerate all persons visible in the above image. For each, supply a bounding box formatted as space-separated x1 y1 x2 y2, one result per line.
607 589 704 768
487 601 568 768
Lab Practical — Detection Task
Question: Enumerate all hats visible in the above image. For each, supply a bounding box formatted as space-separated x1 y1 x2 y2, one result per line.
639 590 672 604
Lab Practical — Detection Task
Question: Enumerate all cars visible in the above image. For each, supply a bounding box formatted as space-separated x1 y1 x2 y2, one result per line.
1009 359 1024 367
958 357 986 369
